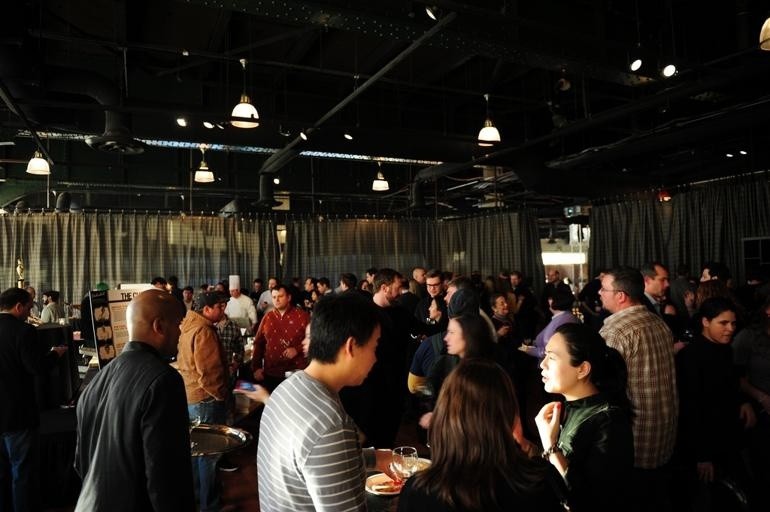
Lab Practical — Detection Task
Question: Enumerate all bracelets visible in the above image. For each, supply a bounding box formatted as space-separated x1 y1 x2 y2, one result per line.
540 443 561 461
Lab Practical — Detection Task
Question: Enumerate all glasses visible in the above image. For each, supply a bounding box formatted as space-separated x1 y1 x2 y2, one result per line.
600 284 629 297
426 282 441 286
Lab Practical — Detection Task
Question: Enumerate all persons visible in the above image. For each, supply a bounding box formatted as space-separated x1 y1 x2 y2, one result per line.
392 358 570 512
257 291 381 512
25 287 40 318
1 286 67 512
151 277 166 292
487 295 518 346
443 269 487 289
699 262 732 297
640 262 670 322
225 274 257 382
178 291 233 512
598 266 679 510
409 266 429 295
38 290 64 325
536 322 635 509
181 285 198 310
420 311 529 453
252 276 283 313
739 261 769 304
368 267 405 451
254 287 310 382
293 276 333 308
480 270 524 297
414 268 446 322
545 268 573 299
683 291 701 312
681 297 757 512
201 284 211 290
520 281 581 357
166 276 186 320
334 269 377 295
70 290 198 512
214 282 223 291
733 299 769 409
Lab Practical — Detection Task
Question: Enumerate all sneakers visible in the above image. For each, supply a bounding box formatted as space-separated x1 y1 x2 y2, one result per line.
217 461 239 472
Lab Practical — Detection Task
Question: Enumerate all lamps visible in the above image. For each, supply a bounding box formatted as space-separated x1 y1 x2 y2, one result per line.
478 118 501 148
760 17 770 51
230 97 260 129
372 173 389 192
26 149 51 176
195 161 215 183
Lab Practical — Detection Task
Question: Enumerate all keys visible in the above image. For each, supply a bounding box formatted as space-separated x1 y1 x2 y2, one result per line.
212 300 245 419
408 290 496 400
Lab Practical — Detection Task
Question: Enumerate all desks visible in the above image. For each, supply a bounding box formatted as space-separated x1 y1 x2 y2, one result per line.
203 447 404 512
30 323 76 410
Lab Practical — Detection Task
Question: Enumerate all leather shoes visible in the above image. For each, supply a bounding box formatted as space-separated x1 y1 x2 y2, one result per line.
220 503 240 512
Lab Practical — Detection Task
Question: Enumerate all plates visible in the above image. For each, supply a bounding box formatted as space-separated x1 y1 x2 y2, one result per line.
189 423 253 457
387 456 433 476
364 473 400 496
517 346 537 351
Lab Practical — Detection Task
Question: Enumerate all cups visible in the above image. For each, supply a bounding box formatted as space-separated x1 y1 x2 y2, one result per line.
391 445 418 478
524 336 532 346
416 382 434 412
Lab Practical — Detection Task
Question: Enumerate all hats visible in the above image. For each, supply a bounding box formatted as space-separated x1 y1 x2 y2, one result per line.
343 273 357 284
228 276 239 289
193 292 225 310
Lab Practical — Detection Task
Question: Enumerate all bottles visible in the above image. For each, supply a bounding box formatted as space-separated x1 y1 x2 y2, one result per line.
234 383 253 417
